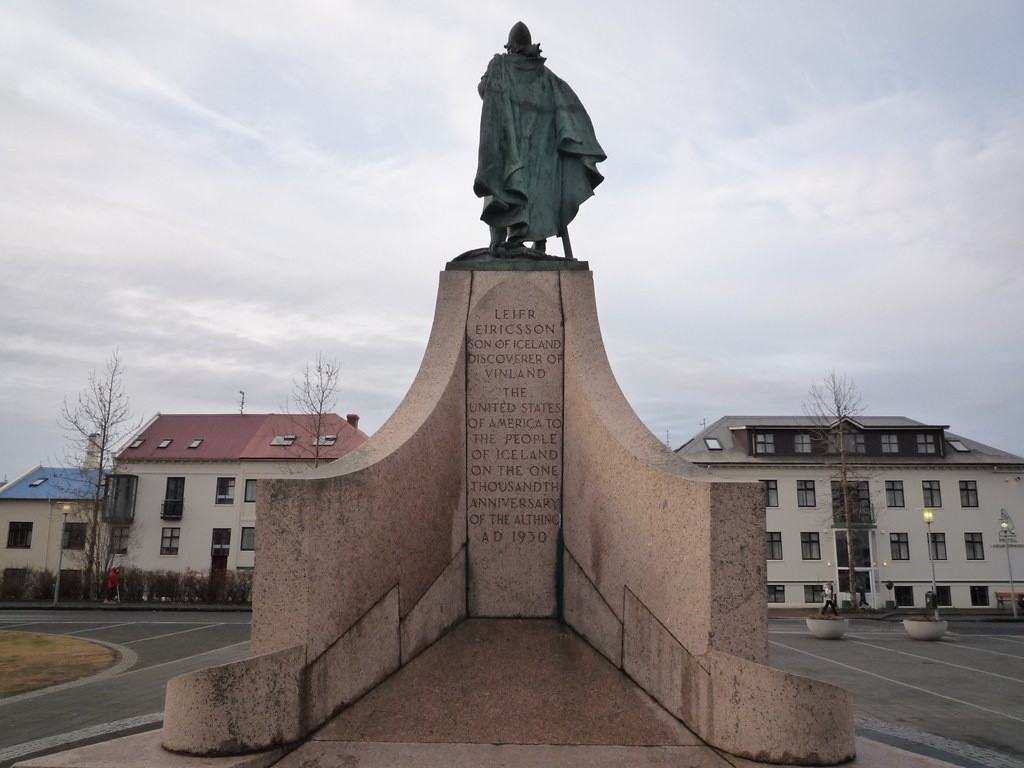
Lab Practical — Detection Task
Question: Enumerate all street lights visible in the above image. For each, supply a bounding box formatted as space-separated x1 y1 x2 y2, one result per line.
52 504 72 606
921 509 940 620
1000 521 1018 618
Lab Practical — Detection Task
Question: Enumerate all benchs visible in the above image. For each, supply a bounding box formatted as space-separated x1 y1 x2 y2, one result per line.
995 592 1022 608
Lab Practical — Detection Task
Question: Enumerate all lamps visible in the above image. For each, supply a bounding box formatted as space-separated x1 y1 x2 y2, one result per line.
1015 477 1020 481
827 560 835 567
873 561 877 567
881 560 887 567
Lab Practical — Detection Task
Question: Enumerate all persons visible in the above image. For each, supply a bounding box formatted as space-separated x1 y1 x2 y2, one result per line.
820 582 839 617
103 566 122 606
858 584 871 609
475 20 606 259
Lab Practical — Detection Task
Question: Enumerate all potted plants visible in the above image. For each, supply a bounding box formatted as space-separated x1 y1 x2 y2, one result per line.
885 580 895 609
842 584 851 609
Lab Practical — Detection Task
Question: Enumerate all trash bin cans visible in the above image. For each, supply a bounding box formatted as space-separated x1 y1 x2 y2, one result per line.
924 590 937 609
